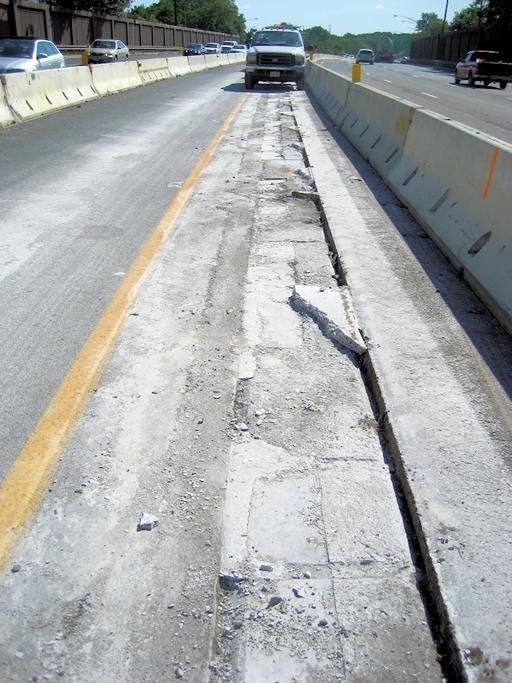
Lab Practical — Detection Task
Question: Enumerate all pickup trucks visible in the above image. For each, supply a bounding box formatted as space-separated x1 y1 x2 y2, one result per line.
454 48 512 89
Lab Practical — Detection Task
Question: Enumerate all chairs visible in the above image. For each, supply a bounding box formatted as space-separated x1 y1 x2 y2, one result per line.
260 37 270 44
26 47 40 55
4 45 23 54
285 38 297 46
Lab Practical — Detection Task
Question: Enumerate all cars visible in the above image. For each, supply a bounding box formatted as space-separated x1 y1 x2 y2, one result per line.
182 39 248 57
0 36 65 74
87 38 130 64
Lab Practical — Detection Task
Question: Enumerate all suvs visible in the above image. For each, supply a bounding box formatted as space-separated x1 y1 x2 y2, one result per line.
244 25 313 91
356 48 375 65
